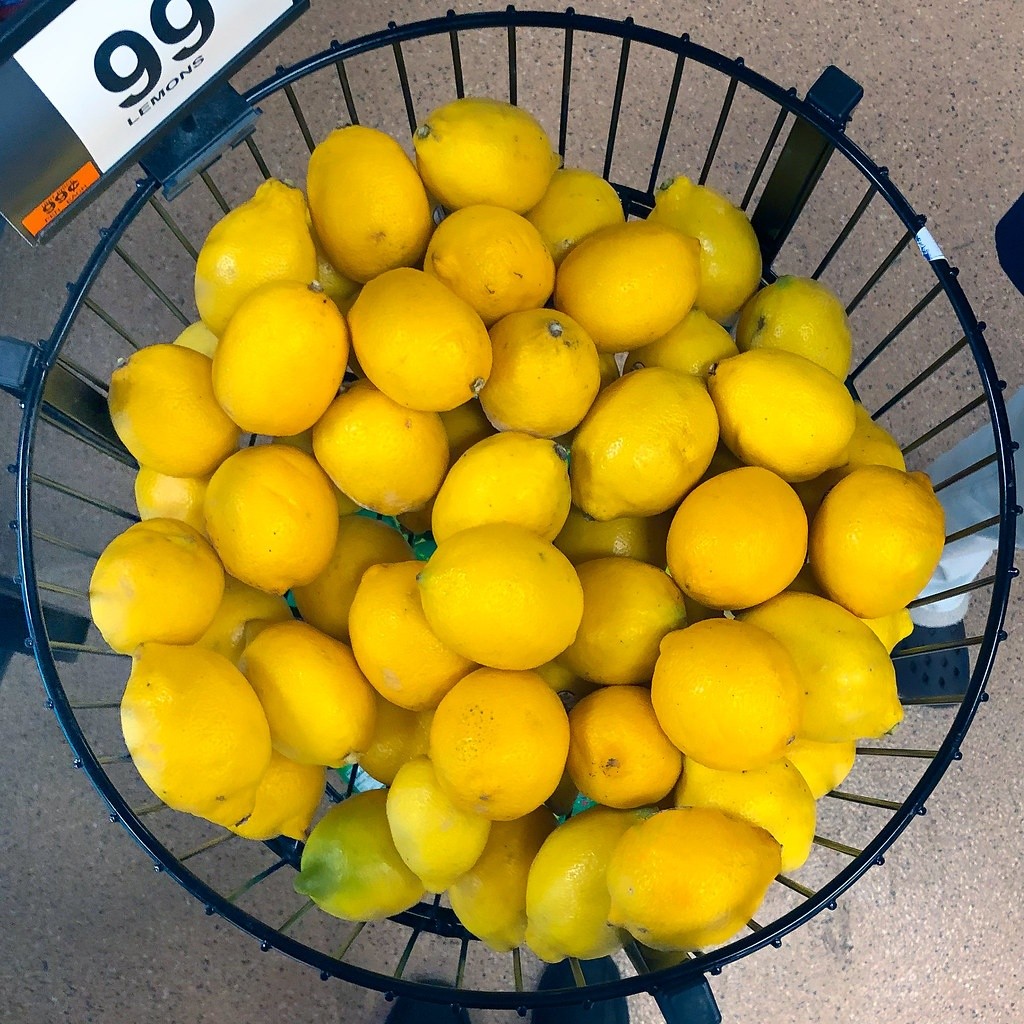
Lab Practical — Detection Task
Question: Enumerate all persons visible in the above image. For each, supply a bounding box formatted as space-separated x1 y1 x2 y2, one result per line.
386 957 634 1024
890 196 1024 703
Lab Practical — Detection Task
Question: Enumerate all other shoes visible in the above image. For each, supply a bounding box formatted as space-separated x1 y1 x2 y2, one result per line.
530 956 631 1023
385 978 472 1024
892 623 972 709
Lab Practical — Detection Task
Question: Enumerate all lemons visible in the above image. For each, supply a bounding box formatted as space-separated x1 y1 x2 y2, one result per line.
90 94 948 964
